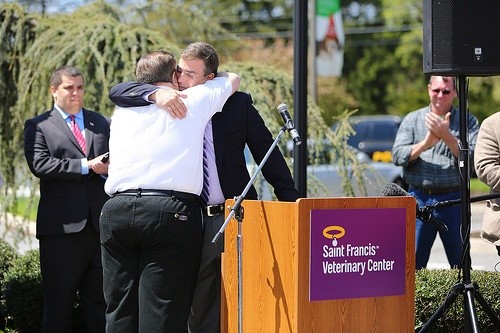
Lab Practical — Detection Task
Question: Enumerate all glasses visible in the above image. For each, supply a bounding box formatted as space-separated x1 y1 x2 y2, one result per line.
176 67 205 81
431 88 451 97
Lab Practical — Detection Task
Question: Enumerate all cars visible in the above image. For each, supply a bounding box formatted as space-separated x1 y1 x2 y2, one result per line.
244 136 405 201
324 115 402 160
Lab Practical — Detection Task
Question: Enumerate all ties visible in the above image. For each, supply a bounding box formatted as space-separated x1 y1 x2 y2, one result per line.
201 131 210 204
68 114 86 156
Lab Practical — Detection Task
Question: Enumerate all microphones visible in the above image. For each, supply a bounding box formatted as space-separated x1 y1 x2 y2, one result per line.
276 104 302 145
382 183 448 232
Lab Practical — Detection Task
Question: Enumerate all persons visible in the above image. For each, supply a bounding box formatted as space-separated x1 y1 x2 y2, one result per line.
23 65 110 333
475 111 500 256
98 50 241 333
390 76 479 270
108 41 301 333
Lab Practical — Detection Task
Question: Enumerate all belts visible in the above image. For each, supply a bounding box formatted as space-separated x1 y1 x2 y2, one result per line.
414 187 460 196
114 189 172 198
205 204 224 218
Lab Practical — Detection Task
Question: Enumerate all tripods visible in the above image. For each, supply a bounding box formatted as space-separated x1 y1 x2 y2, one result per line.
417 77 500 333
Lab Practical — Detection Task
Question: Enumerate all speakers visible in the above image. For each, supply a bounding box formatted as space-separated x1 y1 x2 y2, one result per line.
423 0 500 77
